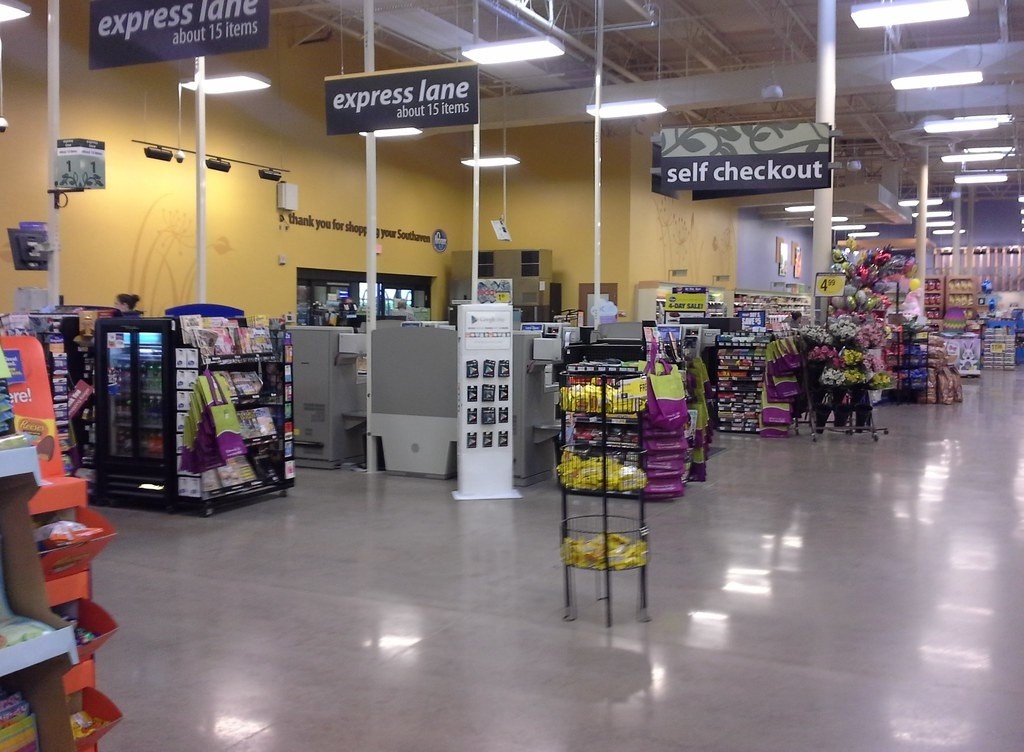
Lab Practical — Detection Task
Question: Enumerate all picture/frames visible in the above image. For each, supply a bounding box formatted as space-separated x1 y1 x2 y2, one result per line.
779 243 789 277
794 246 803 278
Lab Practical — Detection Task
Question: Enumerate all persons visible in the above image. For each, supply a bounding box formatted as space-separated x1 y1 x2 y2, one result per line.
114 293 140 312
790 311 803 328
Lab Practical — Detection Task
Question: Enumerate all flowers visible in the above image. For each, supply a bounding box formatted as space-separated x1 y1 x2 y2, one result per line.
800 312 899 390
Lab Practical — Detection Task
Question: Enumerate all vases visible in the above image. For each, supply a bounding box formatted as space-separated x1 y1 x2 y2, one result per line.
851 387 868 411
833 405 853 427
808 365 824 388
856 404 873 432
812 389 825 411
831 387 847 410
816 404 831 435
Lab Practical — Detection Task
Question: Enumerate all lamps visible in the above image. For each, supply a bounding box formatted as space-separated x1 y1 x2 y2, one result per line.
0 0 32 22
180 70 272 94
359 0 1024 244
144 146 282 181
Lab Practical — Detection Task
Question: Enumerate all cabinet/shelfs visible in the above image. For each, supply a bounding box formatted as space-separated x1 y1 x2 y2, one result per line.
715 334 774 433
450 247 552 322
556 366 642 501
513 331 562 488
945 275 979 319
734 288 815 335
1 298 298 752
925 274 944 319
637 280 730 323
897 329 930 404
551 370 651 627
